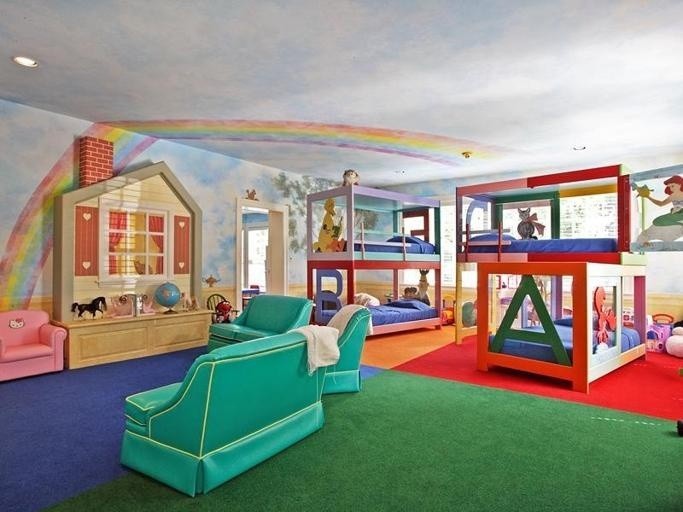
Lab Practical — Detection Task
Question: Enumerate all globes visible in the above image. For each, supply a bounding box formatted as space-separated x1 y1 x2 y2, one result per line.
155 283 181 315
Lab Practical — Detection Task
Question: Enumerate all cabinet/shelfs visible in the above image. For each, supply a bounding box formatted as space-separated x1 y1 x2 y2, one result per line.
45 300 220 373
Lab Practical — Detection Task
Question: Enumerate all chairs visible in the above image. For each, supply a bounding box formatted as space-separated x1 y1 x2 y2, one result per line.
0 308 68 383
114 321 335 498
203 293 314 355
312 302 372 396
205 293 240 324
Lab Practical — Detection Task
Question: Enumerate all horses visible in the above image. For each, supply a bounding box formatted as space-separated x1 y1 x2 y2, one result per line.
71 296 106 320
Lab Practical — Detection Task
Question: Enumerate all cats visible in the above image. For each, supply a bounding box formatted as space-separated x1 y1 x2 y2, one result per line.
343 169 360 186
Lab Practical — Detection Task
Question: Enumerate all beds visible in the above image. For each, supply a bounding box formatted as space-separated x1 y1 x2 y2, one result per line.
443 161 653 398
299 183 446 341
